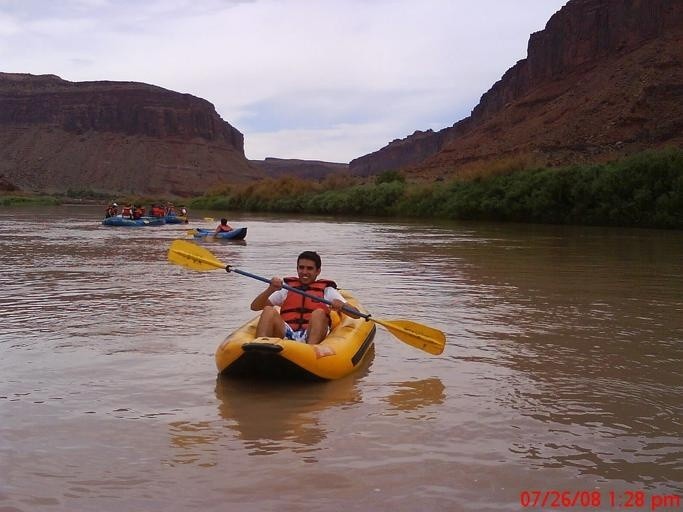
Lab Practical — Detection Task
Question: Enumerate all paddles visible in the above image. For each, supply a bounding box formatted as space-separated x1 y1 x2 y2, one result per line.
166 238 445 356
187 230 215 235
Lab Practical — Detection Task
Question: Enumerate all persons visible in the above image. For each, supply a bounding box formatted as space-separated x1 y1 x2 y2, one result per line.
250 251 359 345
103 200 186 218
214 218 233 234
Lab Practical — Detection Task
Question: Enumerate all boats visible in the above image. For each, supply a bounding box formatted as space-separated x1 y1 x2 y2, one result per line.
100 214 189 226
194 227 247 241
214 291 377 383
